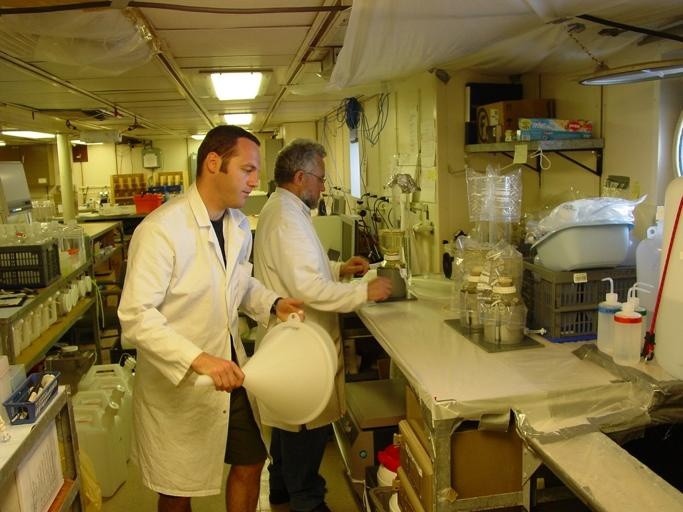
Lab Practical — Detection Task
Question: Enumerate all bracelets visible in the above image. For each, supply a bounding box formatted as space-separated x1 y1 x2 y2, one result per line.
268 296 283 316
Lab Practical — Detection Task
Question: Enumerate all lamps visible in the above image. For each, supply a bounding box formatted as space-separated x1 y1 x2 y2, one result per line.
567 26 683 88
209 71 263 125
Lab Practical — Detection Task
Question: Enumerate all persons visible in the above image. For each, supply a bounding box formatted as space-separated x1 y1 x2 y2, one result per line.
254 135 396 512
113 123 308 512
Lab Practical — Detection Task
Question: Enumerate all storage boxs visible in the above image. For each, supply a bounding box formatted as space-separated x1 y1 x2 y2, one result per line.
477 99 555 145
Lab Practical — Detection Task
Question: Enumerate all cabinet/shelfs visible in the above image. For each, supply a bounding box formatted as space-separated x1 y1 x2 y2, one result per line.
0 203 159 512
335 267 683 512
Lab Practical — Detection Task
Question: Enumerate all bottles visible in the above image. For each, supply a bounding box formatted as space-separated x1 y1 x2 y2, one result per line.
164 192 170 203
629 281 654 357
458 270 524 345
612 287 651 367
596 277 622 355
58 346 83 358
0 200 86 268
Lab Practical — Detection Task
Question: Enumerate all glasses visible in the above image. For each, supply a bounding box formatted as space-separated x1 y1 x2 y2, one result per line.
292 170 326 187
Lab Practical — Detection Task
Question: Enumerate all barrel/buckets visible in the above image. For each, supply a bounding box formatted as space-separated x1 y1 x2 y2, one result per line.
71 357 138 498
653 176 683 380
